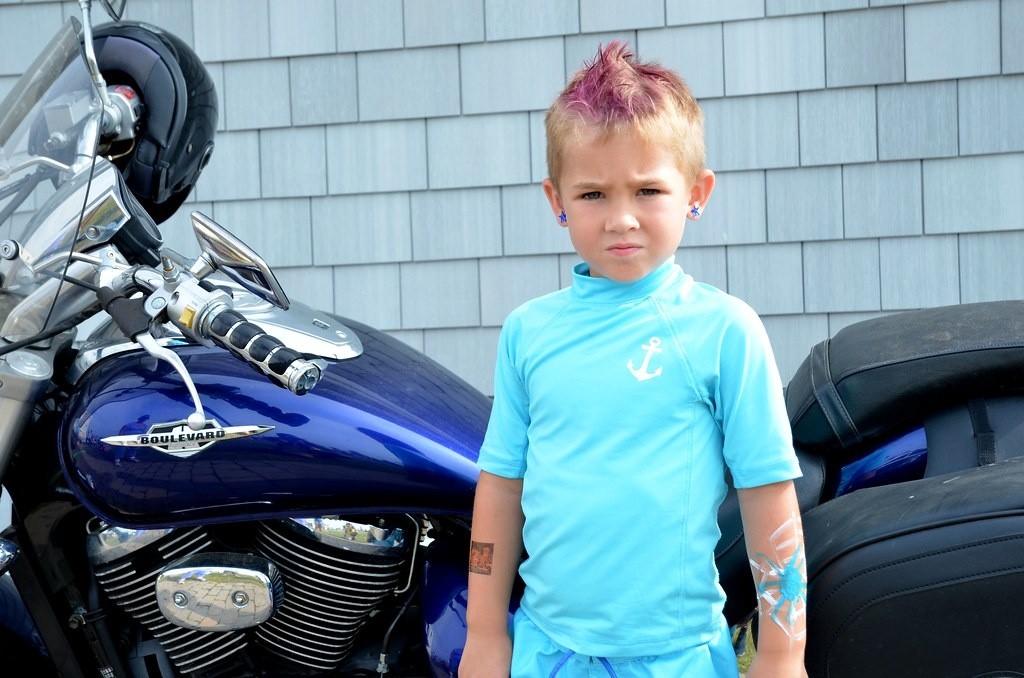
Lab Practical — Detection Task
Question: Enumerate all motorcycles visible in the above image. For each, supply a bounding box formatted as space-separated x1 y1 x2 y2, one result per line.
0 1 1023 678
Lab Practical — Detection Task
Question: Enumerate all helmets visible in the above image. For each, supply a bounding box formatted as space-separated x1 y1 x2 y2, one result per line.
27 18 218 226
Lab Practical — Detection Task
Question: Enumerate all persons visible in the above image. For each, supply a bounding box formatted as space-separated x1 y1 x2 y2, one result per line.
458 41 809 678
